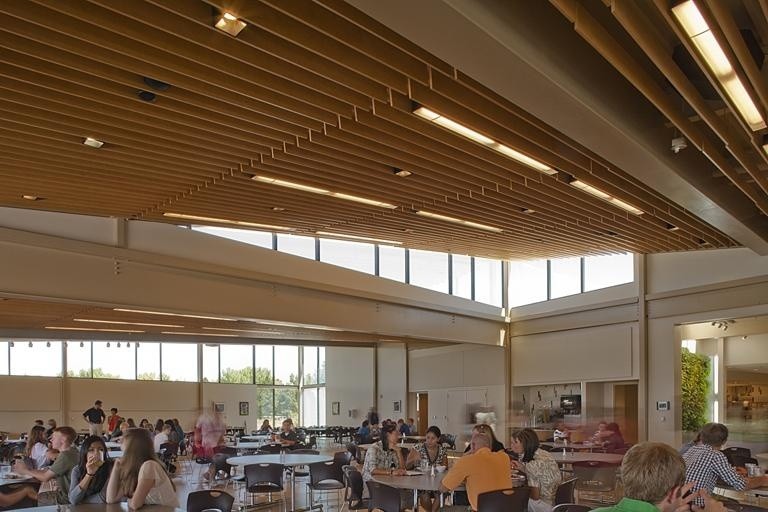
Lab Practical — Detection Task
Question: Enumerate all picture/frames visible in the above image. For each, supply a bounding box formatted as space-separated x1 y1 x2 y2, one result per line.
240 402 249 415
391 400 400 413
332 402 339 415
214 403 225 413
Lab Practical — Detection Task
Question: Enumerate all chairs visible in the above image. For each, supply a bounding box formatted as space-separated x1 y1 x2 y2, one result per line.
0 426 768 512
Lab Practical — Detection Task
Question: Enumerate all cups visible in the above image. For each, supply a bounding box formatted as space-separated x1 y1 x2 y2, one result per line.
744 464 765 477
386 456 447 477
1 464 13 479
279 448 285 458
279 458 285 464
92 448 104 466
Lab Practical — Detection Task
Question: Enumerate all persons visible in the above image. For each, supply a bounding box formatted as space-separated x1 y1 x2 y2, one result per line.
592 422 624 466
234 429 245 438
280 419 297 450
83 402 106 436
14 426 81 490
108 418 184 456
440 424 514 512
361 425 409 512
595 442 723 512
383 418 416 443
195 402 229 479
2 487 38 507
511 420 570 512
261 420 272 430
68 437 127 504
25 418 59 470
356 420 382 464
406 426 447 512
680 422 768 510
108 408 121 441
106 428 179 509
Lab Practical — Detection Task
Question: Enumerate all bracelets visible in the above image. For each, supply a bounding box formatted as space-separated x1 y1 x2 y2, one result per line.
87 473 96 476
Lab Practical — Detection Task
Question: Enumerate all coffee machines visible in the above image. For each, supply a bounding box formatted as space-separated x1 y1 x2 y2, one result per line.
557 394 580 420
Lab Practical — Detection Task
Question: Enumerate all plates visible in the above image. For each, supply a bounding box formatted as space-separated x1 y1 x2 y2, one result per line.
510 469 524 481
